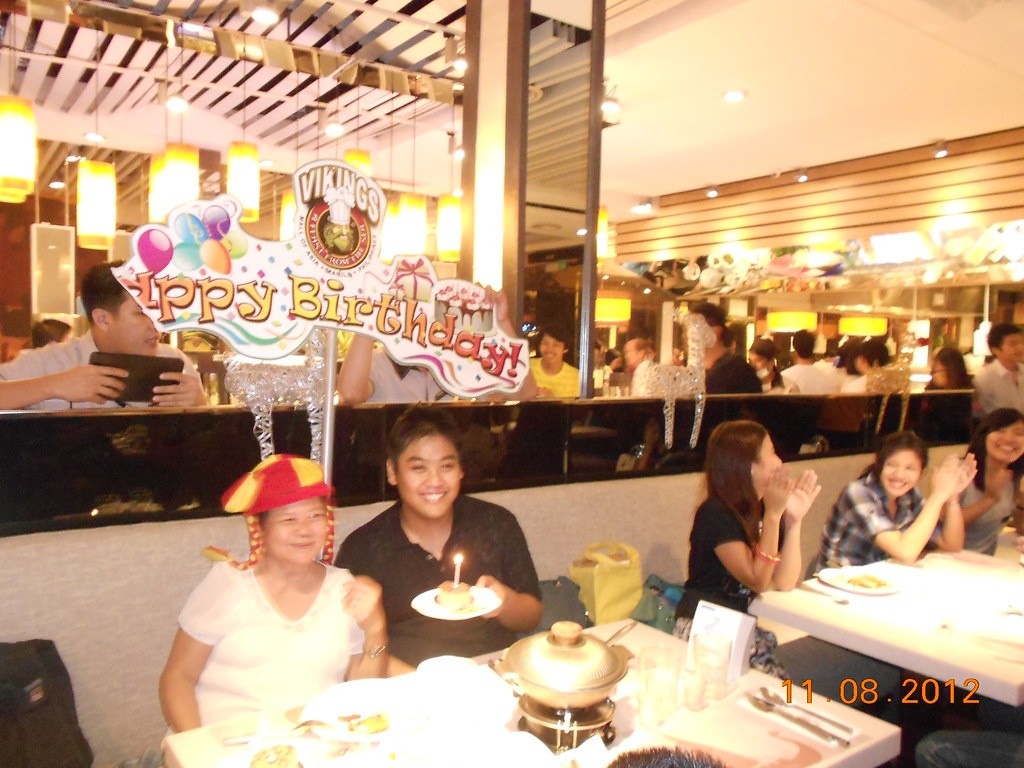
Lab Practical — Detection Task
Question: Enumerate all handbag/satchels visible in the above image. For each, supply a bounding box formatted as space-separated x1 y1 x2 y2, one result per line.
89 351 185 406
566 541 645 627
515 576 589 642
629 574 684 635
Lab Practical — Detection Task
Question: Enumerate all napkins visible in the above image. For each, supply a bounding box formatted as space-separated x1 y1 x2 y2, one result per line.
739 689 859 748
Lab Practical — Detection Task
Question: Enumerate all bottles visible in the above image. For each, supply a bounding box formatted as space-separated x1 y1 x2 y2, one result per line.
994 527 1017 565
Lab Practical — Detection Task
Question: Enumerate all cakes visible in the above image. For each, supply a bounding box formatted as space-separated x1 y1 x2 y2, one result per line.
433 285 495 338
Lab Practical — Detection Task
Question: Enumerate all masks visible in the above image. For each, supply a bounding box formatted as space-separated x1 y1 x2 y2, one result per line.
755 362 771 379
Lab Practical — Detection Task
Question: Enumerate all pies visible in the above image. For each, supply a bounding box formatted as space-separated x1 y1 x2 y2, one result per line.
848 576 887 590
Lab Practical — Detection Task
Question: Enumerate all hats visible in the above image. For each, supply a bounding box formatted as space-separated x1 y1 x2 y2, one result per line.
201 454 337 570
605 349 622 365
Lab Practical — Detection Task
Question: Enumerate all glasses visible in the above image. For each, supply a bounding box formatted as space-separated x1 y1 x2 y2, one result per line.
929 369 946 377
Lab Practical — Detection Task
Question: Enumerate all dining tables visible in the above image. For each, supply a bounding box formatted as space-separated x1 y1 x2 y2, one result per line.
168 619 903 767
749 547 1024 707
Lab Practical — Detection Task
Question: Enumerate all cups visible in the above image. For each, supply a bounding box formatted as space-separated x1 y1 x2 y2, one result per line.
676 632 732 710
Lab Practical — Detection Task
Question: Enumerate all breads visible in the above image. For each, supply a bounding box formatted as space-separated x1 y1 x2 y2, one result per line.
252 707 389 768
436 581 474 610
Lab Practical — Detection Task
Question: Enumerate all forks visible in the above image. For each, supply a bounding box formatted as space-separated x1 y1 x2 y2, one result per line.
745 691 849 748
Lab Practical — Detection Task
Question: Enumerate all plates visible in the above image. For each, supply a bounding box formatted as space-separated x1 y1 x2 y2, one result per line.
822 568 890 597
304 677 400 743
410 586 503 620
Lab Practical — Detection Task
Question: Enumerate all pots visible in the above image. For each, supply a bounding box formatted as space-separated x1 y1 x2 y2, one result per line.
493 619 636 711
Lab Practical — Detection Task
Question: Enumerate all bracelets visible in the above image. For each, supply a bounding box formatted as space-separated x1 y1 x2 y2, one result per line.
364 641 391 657
755 544 780 564
1016 503 1024 511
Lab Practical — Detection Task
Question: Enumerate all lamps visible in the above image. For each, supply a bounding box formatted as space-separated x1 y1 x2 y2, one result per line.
837 317 888 335
765 311 817 333
594 279 633 322
0 0 612 264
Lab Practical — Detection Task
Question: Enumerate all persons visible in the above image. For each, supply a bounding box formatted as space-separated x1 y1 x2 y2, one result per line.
333 406 543 677
684 302 763 394
529 322 580 396
158 453 389 768
780 330 891 392
336 280 539 406
939 408 1024 556
673 420 821 684
748 337 801 394
971 324 1024 421
925 347 975 390
32 318 73 349
803 431 978 768
593 336 655 383
0 260 207 410
608 747 728 768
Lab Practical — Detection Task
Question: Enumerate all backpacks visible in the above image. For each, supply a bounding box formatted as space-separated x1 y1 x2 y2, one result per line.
0 640 93 768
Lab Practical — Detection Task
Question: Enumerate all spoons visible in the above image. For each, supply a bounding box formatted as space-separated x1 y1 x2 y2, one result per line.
760 686 853 734
795 583 848 604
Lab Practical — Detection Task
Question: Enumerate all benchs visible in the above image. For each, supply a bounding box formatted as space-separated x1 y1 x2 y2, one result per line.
0 446 967 768
